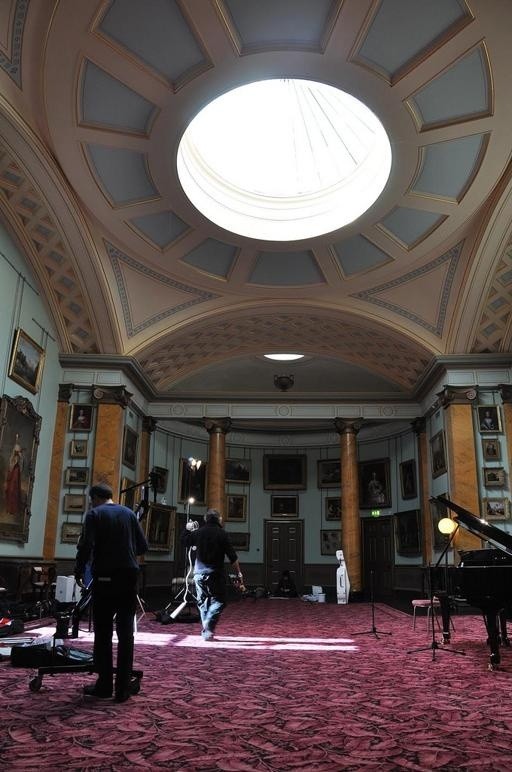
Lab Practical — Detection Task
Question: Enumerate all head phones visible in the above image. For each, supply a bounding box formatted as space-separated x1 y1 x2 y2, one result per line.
204 508 223 526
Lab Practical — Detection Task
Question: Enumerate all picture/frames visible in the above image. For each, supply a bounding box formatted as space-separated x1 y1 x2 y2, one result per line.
224 456 252 485
475 404 511 523
6 327 47 395
428 429 449 480
398 458 418 501
179 457 207 507
122 424 139 472
320 529 343 556
120 476 141 517
359 457 392 510
57 401 96 545
144 502 177 554
427 491 451 551
225 532 250 553
150 464 171 494
0 392 43 546
225 493 247 523
317 458 341 489
262 453 308 491
270 495 299 518
325 496 341 521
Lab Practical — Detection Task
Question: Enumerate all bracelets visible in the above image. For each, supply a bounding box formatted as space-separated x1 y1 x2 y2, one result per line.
236 572 243 577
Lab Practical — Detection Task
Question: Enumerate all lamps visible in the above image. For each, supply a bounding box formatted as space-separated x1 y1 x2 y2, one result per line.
434 517 459 567
181 456 201 525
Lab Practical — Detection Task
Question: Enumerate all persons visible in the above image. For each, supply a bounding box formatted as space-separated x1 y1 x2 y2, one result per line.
76 409 87 428
367 471 385 504
178 509 244 642
76 442 81 452
74 484 149 702
10 431 25 471
5 454 22 515
481 409 498 430
273 569 298 597
156 476 164 489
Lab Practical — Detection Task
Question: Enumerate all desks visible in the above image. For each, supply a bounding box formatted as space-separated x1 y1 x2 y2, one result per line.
140 561 149 603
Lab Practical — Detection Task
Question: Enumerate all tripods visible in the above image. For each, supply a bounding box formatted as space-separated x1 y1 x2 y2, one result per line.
407 562 466 661
113 593 147 633
352 568 391 639
161 465 198 614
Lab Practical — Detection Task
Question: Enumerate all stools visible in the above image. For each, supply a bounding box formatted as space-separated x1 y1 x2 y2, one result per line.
410 599 457 639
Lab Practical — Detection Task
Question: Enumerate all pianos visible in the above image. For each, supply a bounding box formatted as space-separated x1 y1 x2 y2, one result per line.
427 495 512 663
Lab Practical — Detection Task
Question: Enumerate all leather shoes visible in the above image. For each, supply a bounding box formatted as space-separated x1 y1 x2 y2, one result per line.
114 680 141 704
84 685 113 698
